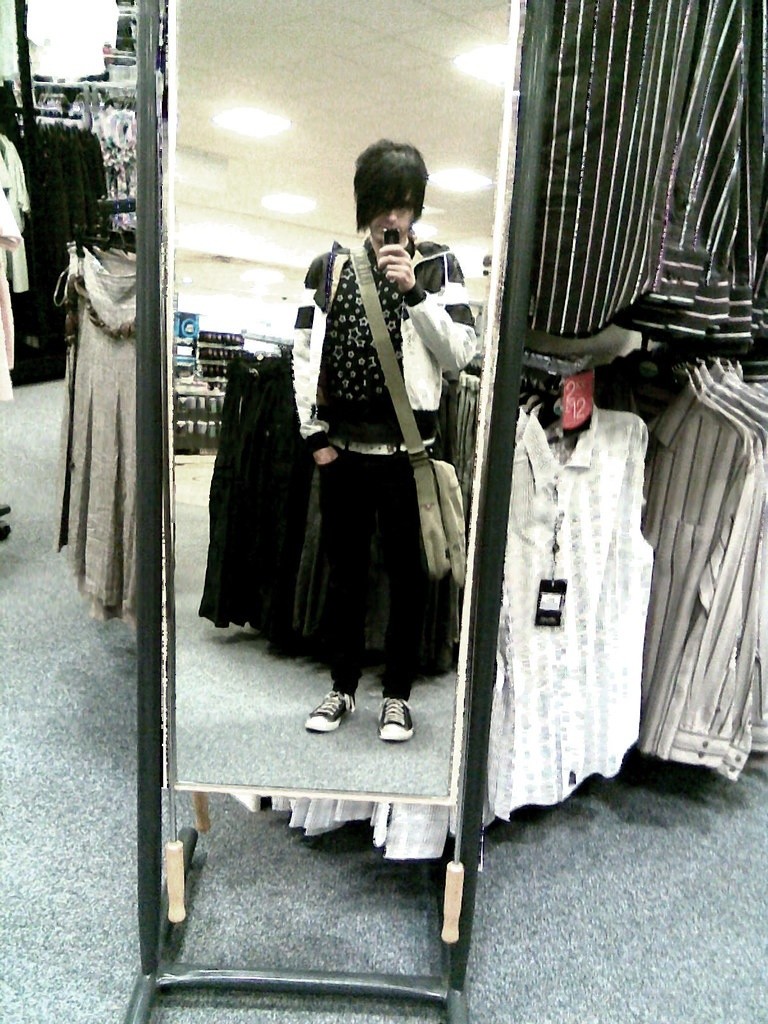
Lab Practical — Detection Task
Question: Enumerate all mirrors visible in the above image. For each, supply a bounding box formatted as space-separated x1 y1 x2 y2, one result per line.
124 0 555 1024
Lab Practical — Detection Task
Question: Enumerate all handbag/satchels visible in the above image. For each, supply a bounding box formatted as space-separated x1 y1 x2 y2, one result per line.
415 457 466 587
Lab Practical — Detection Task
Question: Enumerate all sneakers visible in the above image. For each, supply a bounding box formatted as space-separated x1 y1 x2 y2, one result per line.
304 690 355 731
376 698 414 742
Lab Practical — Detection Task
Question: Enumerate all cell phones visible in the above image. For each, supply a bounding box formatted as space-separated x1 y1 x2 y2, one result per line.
383 228 400 247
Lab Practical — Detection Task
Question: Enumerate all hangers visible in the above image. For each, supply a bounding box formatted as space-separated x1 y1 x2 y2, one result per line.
28 75 138 145
65 197 137 256
518 331 767 443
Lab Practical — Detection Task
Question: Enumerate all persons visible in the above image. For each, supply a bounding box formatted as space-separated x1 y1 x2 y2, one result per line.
290 136 476 746
